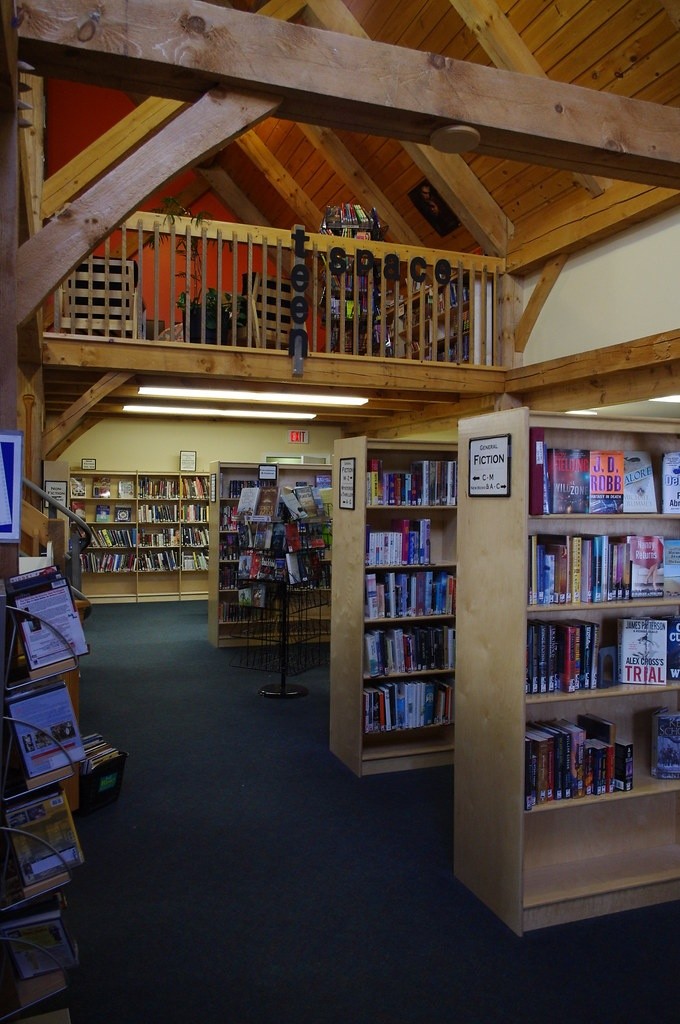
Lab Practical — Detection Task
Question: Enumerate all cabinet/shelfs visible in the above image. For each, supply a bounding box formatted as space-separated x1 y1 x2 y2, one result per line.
451 406 680 939
69 470 211 605
329 435 457 781
1 591 89 1024
205 458 332 652
316 202 474 365
225 509 331 702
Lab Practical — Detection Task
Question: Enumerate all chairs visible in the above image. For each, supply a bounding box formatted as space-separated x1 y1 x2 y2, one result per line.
228 272 294 351
61 256 142 341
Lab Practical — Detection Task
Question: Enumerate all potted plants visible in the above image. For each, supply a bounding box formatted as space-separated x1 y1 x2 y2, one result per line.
143 185 253 347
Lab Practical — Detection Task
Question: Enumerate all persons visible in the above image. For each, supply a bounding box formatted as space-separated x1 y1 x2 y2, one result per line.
644 564 660 592
412 184 458 234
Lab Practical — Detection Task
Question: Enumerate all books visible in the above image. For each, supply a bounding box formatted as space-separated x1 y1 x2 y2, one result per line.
522 423 680 812
386 270 469 365
313 205 387 364
219 468 334 620
364 447 459 734
2 566 123 987
72 473 211 575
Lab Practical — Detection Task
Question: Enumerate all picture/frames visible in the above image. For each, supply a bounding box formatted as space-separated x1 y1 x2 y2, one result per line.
81 458 97 470
179 450 197 472
